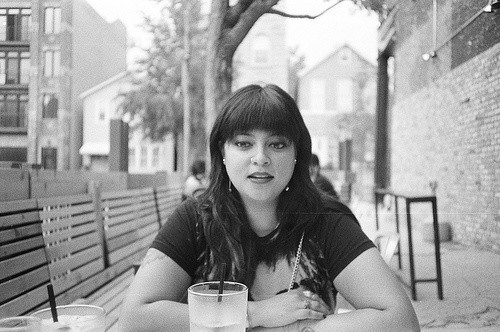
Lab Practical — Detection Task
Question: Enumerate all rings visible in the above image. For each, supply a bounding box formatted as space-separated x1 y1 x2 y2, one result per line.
301 298 312 310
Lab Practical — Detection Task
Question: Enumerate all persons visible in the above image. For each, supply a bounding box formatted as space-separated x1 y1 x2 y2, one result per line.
309 155 340 201
181 159 208 201
121 83 422 332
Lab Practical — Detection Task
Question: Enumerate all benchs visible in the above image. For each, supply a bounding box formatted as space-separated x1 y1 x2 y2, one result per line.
0 184 184 332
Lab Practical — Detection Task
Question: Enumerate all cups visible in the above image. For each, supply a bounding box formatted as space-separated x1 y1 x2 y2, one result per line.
29 304 106 332
187 281 248 332
0 317 42 332
